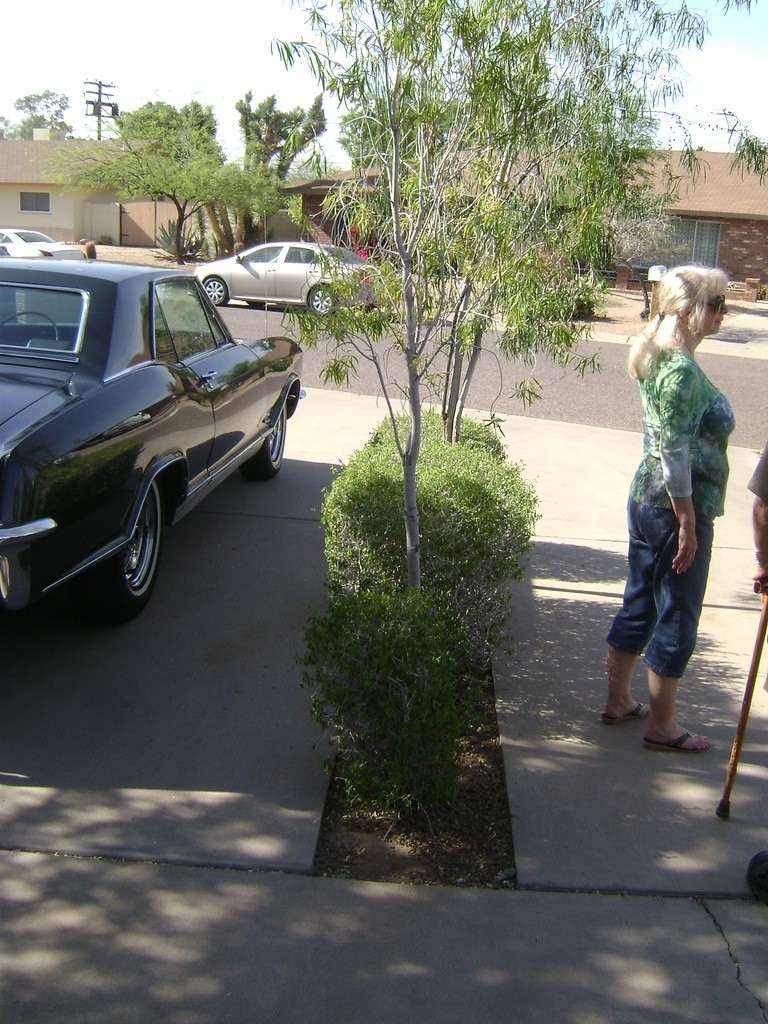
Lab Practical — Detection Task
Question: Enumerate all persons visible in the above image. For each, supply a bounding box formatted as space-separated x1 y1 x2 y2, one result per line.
598 264 737 753
745 439 768 598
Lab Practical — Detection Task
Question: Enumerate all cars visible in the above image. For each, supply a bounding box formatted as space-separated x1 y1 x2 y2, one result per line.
0 229 88 262
194 240 381 319
1 255 304 632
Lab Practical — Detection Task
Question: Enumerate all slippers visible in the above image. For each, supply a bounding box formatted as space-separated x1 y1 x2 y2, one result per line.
642 732 710 754
600 701 648 724
